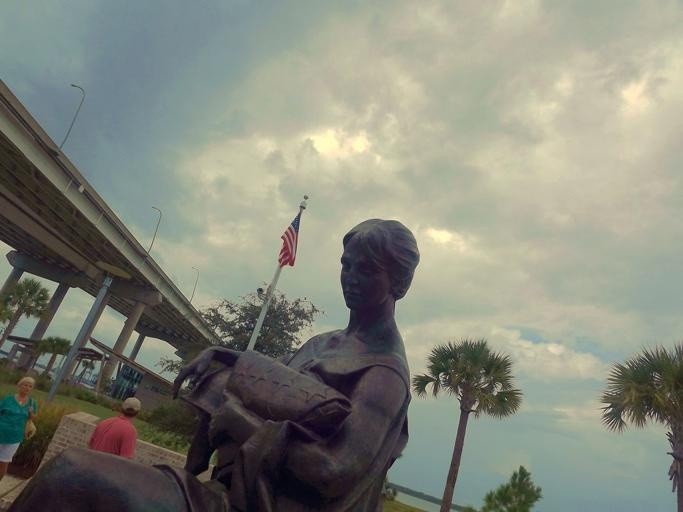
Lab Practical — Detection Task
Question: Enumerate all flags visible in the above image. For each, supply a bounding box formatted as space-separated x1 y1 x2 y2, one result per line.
276 212 299 267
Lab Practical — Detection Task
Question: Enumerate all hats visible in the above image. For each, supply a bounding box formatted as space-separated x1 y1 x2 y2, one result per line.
122 397 140 413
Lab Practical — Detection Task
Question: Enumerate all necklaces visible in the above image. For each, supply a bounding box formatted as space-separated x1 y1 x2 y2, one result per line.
18 400 22 404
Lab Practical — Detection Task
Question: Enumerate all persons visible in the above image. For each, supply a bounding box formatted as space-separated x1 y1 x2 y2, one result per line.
5 218 421 512
0 375 38 480
86 397 143 462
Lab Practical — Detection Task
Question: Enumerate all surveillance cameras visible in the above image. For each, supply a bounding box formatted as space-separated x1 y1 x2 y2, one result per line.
257 288 266 293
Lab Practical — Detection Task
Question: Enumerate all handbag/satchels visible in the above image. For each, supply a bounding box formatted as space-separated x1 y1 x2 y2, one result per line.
25 418 38 440
